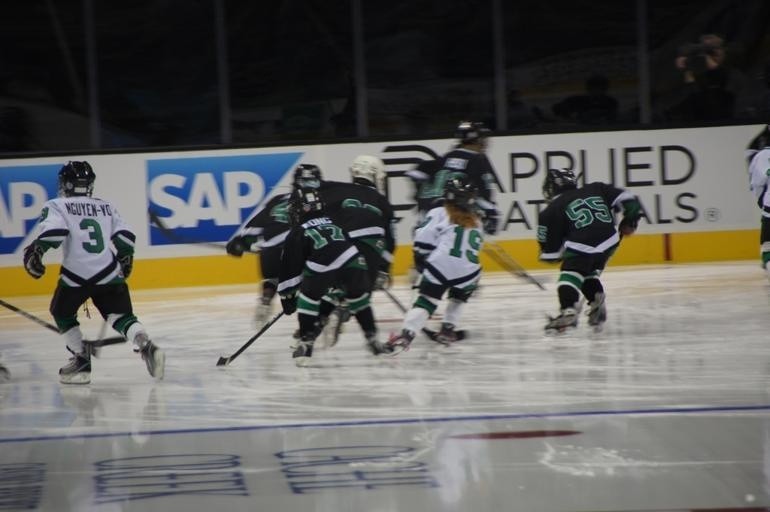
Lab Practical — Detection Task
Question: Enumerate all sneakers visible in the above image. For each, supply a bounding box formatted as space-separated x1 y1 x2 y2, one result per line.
586 301 607 325
437 320 464 341
138 339 157 376
292 337 313 358
59 348 91 374
383 329 415 349
323 309 342 347
367 337 384 355
543 309 578 329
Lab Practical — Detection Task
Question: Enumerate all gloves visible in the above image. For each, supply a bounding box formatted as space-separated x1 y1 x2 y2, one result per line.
116 253 133 278
23 244 46 279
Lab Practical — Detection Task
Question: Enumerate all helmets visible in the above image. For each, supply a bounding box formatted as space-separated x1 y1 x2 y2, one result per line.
454 118 491 141
349 155 386 185
443 177 478 211
542 167 577 199
293 163 321 188
58 161 96 197
288 187 325 229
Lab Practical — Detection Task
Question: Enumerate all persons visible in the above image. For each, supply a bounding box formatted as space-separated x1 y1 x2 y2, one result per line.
277 187 390 369
407 118 501 286
389 176 485 356
287 154 396 350
23 160 167 385
225 163 323 329
747 137 770 271
536 167 643 339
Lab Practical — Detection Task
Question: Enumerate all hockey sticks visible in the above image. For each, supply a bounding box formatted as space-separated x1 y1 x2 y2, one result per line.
0 298 127 348
379 286 458 346
215 310 286 367
